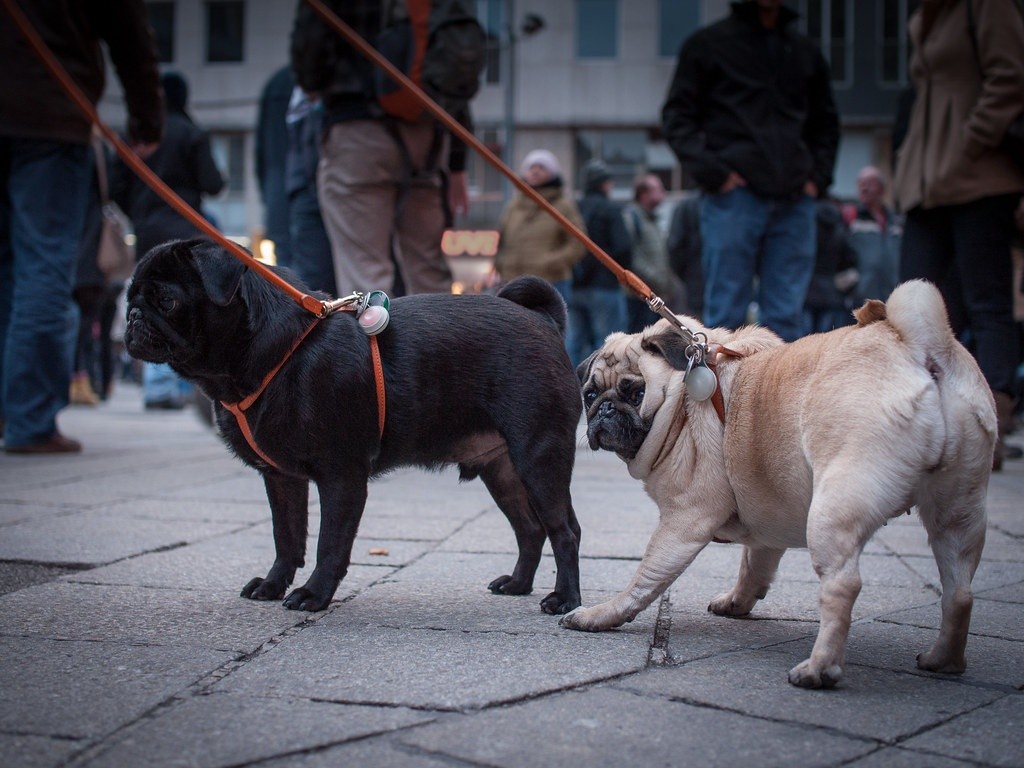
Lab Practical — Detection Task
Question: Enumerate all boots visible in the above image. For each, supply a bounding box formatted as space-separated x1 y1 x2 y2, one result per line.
991 390 1017 472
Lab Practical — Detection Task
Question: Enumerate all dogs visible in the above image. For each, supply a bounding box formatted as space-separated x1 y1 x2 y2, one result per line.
124 236 584 616
555 280 1000 692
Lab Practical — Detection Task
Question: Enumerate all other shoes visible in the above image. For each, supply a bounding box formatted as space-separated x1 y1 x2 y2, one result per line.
146 392 184 409
69 378 107 405
5 429 82 454
1004 430 1024 453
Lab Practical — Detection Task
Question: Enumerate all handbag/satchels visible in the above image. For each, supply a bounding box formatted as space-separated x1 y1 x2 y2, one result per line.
98 206 133 275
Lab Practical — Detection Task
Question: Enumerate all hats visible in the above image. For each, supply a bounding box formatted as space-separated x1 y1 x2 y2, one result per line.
579 158 615 181
520 148 563 179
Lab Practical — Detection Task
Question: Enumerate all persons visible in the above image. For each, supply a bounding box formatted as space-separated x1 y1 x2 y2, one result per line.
64 70 224 411
660 1 842 341
494 148 705 371
893 0 1024 472
803 166 894 332
288 0 474 299
0 0 167 454
253 58 336 293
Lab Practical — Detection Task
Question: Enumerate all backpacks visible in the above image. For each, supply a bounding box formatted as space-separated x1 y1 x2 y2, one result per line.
374 0 488 126
